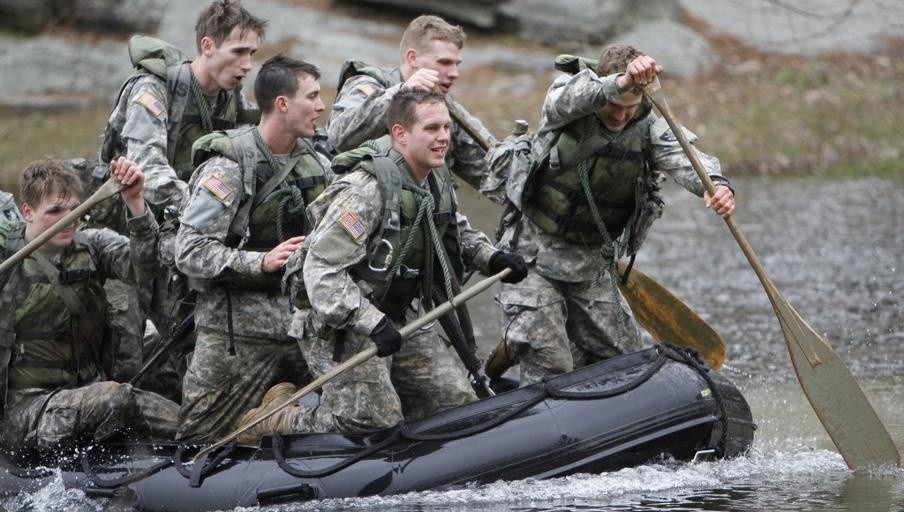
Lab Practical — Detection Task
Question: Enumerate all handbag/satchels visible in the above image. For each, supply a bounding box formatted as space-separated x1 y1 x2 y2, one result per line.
481 119 539 211
68 157 119 224
281 247 309 310
157 218 185 298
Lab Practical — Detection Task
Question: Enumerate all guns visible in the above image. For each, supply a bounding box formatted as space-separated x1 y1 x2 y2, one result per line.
124 309 196 384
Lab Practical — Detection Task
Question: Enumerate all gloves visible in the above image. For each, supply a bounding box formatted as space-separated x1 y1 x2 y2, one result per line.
489 251 528 284
370 316 402 358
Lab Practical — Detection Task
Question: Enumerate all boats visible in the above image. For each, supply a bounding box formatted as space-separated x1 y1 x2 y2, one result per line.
1 343 755 512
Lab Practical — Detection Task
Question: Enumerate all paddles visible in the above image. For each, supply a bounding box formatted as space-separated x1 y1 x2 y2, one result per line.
637 76 900 473
194 267 512 463
447 101 727 370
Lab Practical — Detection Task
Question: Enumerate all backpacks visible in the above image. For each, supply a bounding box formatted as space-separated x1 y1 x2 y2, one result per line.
0 190 103 375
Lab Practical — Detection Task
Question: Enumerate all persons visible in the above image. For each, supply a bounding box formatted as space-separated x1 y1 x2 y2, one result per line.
95 1 271 405
322 13 509 208
235 84 531 446
483 41 736 391
1 156 181 471
173 50 334 455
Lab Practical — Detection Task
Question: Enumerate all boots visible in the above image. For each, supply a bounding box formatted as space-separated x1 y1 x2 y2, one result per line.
238 382 298 443
486 337 521 378
